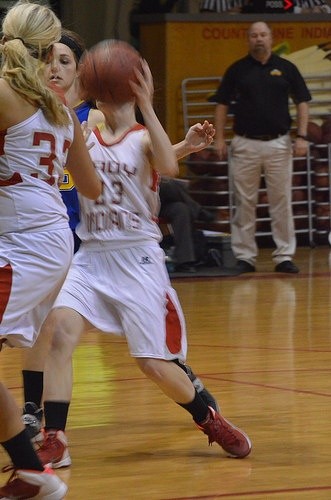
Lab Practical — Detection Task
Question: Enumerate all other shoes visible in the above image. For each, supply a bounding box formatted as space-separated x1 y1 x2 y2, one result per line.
227 260 255 276
197 207 218 221
275 261 299 273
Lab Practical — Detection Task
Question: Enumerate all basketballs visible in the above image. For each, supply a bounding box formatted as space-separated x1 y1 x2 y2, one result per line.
80 48 141 105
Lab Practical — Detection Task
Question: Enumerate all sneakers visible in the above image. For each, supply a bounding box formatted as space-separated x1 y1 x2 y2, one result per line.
0 464 68 500
195 405 252 457
35 429 71 467
173 359 220 413
20 401 44 440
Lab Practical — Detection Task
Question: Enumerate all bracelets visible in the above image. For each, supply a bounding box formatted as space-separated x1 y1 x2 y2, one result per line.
296 135 310 141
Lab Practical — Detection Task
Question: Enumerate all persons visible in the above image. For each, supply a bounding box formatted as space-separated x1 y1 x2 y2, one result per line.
31 57 253 471
17 27 100 453
199 0 331 14
156 181 218 275
212 20 313 276
0 3 105 500
